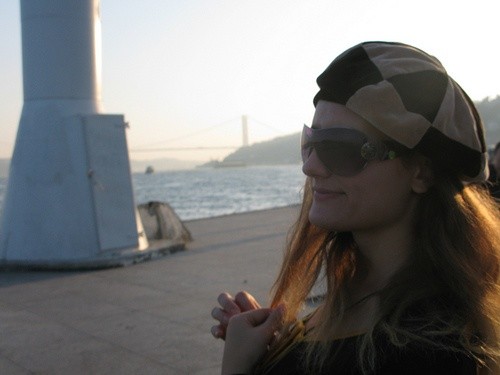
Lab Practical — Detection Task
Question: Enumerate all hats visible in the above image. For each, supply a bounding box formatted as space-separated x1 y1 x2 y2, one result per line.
313 41 489 183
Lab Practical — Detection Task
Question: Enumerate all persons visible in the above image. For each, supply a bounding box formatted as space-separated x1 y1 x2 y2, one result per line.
211 41 500 375
486 142 500 199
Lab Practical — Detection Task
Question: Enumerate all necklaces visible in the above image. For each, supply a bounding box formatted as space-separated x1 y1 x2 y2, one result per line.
303 284 389 337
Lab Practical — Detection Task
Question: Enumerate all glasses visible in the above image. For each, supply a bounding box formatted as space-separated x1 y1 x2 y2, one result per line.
301 124 411 177
